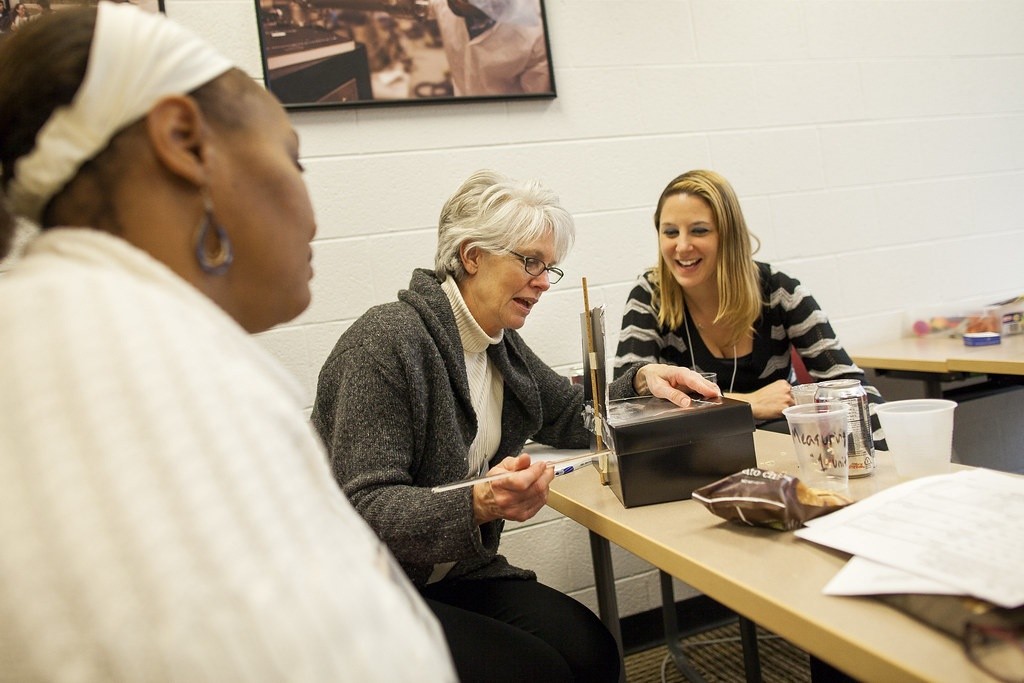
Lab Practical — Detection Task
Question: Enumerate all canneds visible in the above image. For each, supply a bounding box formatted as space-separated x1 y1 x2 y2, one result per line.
814 379 874 479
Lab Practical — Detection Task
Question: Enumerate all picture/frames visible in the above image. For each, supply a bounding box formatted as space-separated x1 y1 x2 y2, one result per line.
254 0 559 113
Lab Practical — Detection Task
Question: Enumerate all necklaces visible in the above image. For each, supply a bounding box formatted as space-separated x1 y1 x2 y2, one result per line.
689 301 705 332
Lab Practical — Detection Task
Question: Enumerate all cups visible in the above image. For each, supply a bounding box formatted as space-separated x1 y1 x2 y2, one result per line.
789 383 818 405
873 399 958 476
782 403 848 496
700 373 717 383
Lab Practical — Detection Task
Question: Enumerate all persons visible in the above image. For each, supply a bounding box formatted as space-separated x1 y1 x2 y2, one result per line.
309 168 723 683
0 2 459 683
611 168 888 453
429 0 551 97
0 0 57 33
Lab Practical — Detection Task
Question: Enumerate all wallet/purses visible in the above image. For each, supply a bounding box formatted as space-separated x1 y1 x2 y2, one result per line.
870 594 1024 683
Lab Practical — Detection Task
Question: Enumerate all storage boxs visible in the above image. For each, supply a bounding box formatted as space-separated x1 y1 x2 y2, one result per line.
589 394 757 509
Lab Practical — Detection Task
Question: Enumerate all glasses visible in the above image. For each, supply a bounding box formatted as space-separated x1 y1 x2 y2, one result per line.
501 247 564 284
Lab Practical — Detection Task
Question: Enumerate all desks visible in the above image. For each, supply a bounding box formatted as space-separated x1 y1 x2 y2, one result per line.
515 428 1024 683
837 333 1024 403
